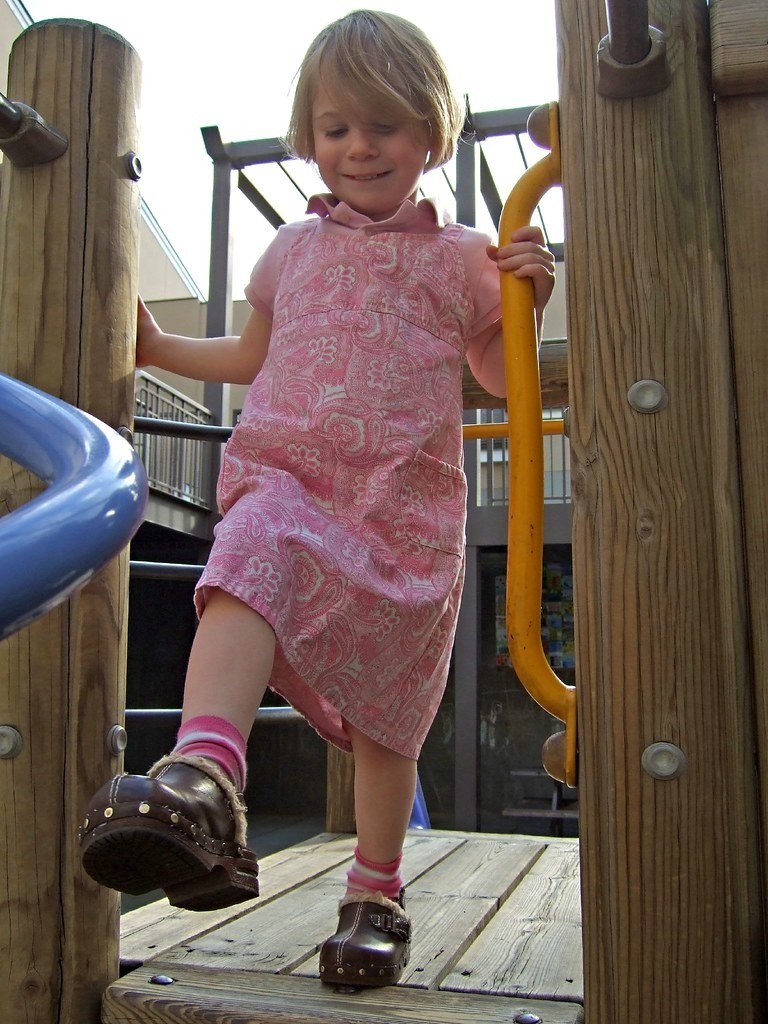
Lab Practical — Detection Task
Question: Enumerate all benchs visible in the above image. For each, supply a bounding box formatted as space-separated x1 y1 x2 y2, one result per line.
522 796 579 808
501 807 578 820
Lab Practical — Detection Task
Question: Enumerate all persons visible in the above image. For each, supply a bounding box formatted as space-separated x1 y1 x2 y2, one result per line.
78 9 555 986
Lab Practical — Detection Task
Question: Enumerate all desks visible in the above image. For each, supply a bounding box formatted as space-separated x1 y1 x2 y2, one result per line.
510 766 577 835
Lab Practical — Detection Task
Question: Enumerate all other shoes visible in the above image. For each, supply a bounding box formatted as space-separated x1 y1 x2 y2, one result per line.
320 887 411 986
80 754 258 913
